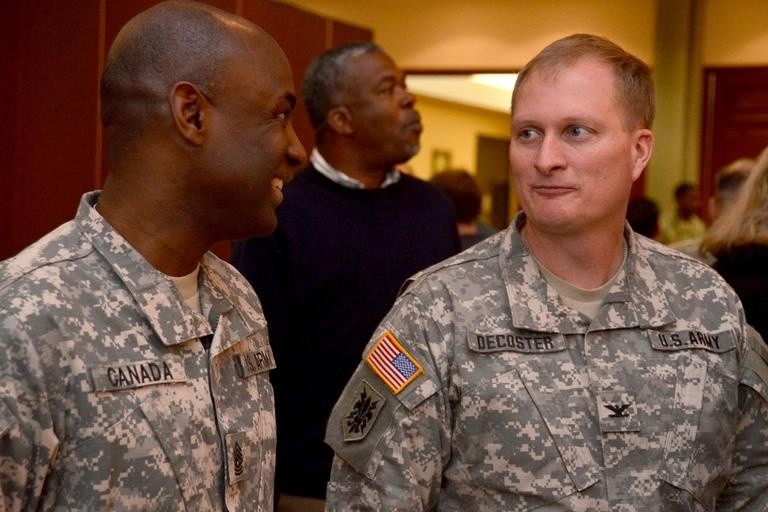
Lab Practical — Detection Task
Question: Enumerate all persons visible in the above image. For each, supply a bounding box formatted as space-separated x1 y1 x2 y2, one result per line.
320 35 767 510
626 147 768 344
0 1 309 509
426 168 499 251
231 39 464 512
